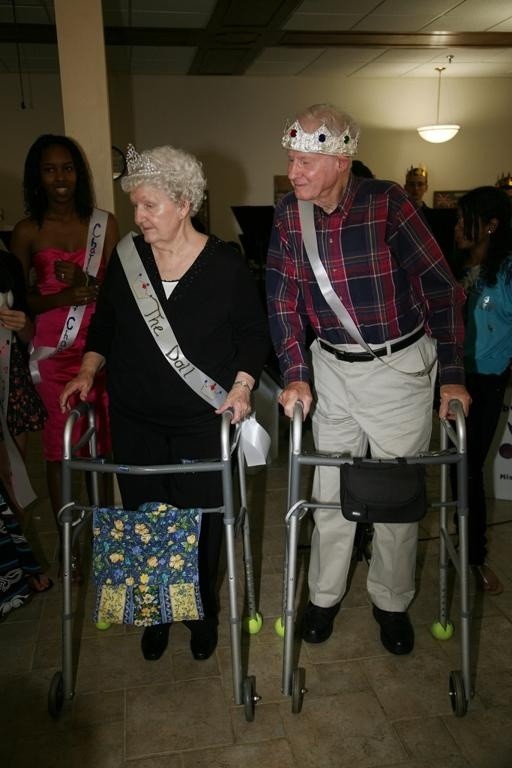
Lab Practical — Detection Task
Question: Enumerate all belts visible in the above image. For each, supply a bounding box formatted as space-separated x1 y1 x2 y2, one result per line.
316 326 426 362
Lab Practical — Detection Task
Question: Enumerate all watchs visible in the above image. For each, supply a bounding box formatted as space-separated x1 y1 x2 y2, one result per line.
233 379 251 394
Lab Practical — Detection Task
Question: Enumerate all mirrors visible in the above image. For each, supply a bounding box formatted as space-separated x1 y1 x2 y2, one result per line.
112 146 127 181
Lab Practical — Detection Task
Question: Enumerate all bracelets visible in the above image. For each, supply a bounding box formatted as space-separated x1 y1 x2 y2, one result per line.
83 272 89 288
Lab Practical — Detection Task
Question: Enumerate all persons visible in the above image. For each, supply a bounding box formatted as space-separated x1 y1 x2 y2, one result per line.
59 143 270 663
9 133 120 587
0 205 55 623
251 190 288 267
267 106 473 657
351 159 511 594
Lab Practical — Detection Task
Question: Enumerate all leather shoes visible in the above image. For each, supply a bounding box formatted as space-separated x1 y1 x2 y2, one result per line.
141 623 169 660
191 615 219 660
373 602 414 654
299 599 340 643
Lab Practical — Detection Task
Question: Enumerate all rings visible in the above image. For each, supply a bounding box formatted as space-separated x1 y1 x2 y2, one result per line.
84 296 87 302
60 273 65 279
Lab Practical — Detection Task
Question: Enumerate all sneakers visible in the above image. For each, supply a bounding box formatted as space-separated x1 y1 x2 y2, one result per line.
473 564 503 596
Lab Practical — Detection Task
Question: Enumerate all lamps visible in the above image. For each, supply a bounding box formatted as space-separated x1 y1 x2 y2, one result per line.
417 68 460 143
